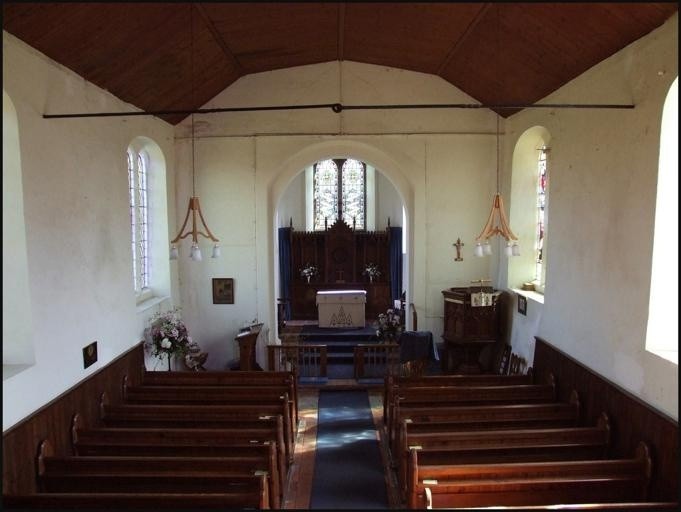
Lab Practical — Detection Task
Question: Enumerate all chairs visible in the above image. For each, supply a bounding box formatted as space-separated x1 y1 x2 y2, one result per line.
402 330 438 372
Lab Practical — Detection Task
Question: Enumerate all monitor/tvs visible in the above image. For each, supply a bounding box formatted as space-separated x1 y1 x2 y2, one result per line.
470 286 494 294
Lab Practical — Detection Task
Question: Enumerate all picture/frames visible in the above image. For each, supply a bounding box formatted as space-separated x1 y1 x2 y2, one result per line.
518 295 526 316
212 277 234 305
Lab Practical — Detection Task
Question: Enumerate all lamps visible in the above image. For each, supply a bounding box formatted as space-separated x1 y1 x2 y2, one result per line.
169 113 221 262
475 114 521 258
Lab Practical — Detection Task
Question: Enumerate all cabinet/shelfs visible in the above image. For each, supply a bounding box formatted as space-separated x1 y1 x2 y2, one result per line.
315 291 368 330
441 287 507 367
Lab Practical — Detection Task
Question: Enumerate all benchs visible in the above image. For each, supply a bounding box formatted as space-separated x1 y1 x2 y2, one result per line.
10 368 300 512
382 361 681 512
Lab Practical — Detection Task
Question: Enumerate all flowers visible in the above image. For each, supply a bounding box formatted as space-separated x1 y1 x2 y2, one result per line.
144 309 191 358
362 262 382 278
372 309 403 341
301 263 318 278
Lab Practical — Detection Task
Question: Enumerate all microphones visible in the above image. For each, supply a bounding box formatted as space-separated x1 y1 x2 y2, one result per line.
252 318 258 325
